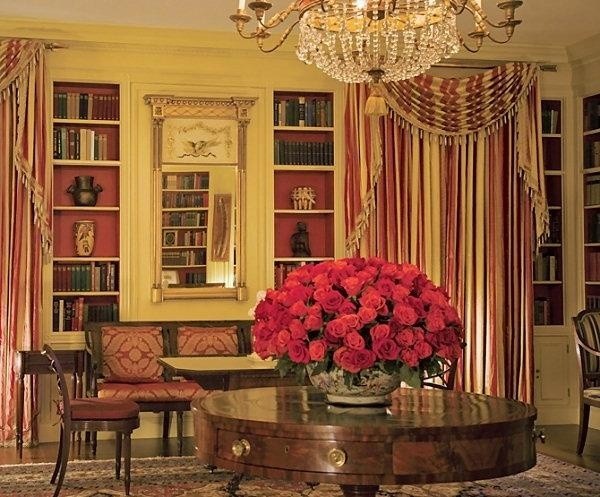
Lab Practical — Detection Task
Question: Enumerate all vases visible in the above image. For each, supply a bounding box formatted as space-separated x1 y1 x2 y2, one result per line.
305 355 407 407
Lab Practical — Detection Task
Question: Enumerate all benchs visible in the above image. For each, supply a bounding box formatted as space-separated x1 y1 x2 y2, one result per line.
83 317 257 455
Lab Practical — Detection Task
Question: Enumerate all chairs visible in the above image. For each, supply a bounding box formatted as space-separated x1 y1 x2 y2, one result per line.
41 345 141 497
571 308 600 457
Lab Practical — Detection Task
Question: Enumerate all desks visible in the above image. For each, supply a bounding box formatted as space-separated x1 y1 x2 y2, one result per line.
157 357 292 473
189 386 537 495
12 349 85 461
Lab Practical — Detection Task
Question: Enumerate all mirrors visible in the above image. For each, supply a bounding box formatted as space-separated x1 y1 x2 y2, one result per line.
151 164 248 304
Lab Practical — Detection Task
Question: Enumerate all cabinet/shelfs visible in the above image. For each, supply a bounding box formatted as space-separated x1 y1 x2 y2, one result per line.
534 92 600 406
160 171 217 286
46 69 129 346
268 84 345 287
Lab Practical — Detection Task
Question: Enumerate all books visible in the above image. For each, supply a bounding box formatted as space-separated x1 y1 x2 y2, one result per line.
542 108 560 134
532 209 564 326
274 261 325 291
52 90 121 162
162 173 207 288
583 104 600 313
52 263 119 332
274 93 334 166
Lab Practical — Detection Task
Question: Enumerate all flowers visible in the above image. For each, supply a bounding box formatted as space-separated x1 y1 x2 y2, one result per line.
250 256 466 391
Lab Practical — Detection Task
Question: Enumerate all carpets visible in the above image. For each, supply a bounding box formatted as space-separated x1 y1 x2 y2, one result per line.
0 448 599 497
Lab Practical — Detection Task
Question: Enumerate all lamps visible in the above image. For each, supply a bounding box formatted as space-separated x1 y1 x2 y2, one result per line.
230 1 526 89
